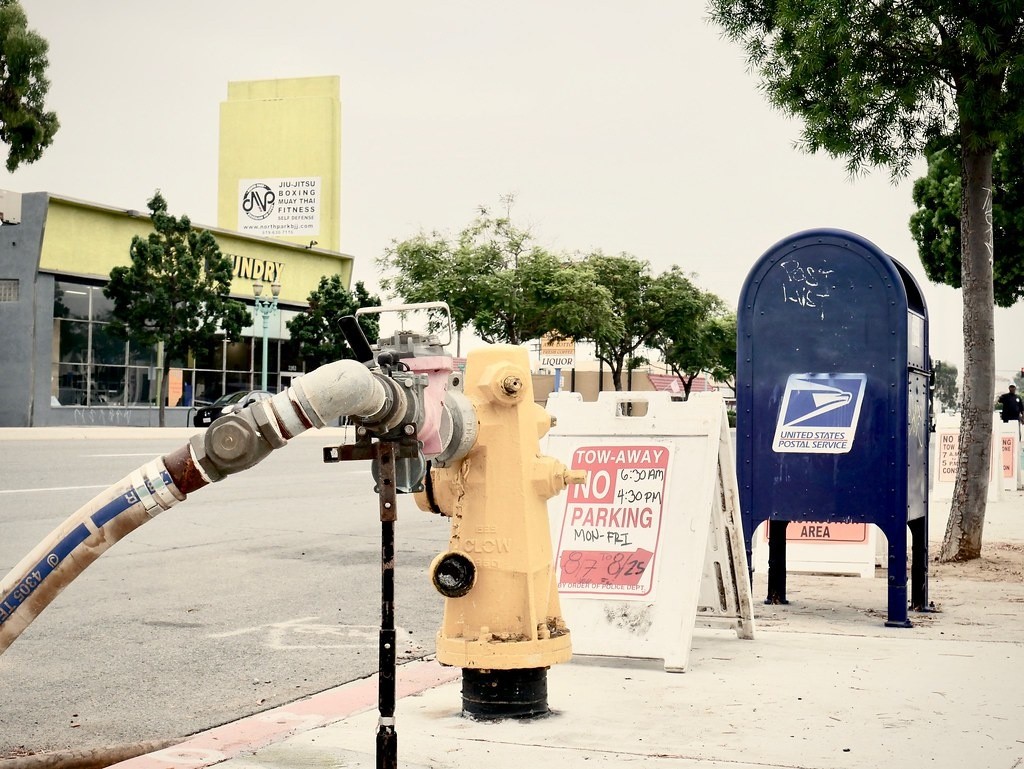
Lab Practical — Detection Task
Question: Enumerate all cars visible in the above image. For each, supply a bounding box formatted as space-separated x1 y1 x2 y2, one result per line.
194 390 276 427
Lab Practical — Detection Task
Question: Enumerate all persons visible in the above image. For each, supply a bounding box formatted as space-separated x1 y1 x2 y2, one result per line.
998 385 1024 441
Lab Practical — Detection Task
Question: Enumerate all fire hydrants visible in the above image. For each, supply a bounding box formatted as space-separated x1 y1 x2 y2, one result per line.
414 343 591 717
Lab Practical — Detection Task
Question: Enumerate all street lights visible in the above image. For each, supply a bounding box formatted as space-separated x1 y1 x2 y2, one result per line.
252 277 281 390
